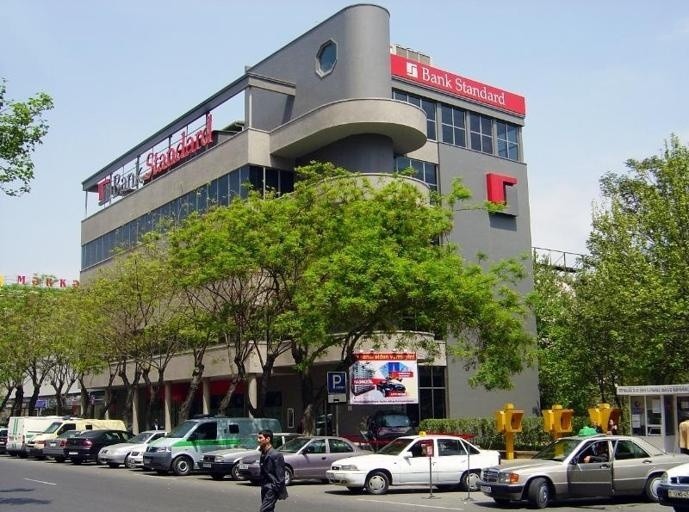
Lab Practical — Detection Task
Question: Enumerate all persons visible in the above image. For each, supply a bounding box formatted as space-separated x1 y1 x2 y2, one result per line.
605 419 618 436
257 430 285 512
583 443 608 462
678 410 689 455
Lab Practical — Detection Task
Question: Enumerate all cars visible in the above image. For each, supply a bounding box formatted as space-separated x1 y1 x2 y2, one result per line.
42 429 172 470
656 461 689 512
477 434 688 510
324 431 502 495
196 432 375 486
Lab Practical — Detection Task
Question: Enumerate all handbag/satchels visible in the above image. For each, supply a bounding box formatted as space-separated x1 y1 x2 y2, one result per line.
278 487 288 501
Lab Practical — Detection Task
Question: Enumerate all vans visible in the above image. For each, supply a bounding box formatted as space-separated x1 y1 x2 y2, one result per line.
142 418 282 477
24 419 127 461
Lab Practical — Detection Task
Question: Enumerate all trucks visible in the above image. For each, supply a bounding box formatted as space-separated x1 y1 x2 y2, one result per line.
6 415 83 457
